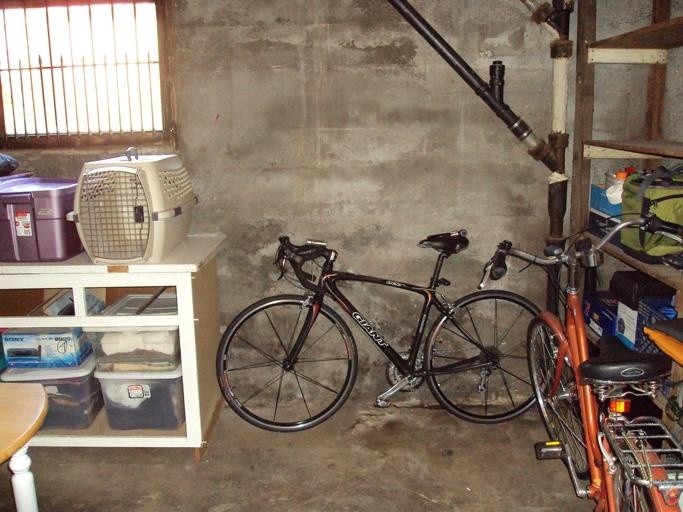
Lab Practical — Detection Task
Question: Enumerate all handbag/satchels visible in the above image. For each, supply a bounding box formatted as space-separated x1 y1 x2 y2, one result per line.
620 165 682 264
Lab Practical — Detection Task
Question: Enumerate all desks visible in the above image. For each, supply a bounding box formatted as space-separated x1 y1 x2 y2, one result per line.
1 381 50 511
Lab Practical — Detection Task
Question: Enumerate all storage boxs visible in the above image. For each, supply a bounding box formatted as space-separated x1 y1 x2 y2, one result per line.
0 176 86 262
0 352 104 429
1 285 105 367
95 364 185 432
82 291 179 371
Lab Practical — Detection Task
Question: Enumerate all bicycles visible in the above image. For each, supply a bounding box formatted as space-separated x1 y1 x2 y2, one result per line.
477 209 683 512
215 230 558 432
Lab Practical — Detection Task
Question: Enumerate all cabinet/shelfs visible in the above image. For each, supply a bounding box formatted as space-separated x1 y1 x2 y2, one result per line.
559 0 682 464
0 235 227 463
